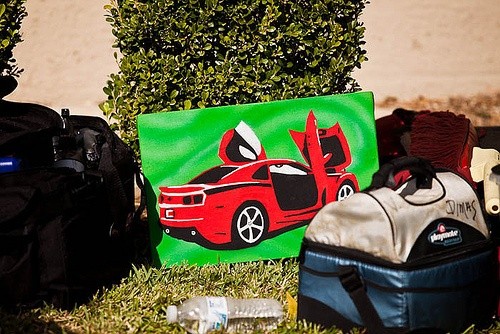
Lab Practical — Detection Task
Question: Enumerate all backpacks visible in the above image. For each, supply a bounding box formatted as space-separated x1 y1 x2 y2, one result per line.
0 99 146 309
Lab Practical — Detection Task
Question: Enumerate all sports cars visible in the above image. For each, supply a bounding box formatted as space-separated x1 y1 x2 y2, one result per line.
156 109 362 251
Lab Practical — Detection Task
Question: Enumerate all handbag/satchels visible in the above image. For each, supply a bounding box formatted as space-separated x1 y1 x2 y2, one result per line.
296 156 499 332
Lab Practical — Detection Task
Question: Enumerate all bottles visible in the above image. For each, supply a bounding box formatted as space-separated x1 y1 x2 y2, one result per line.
165 295 284 333
